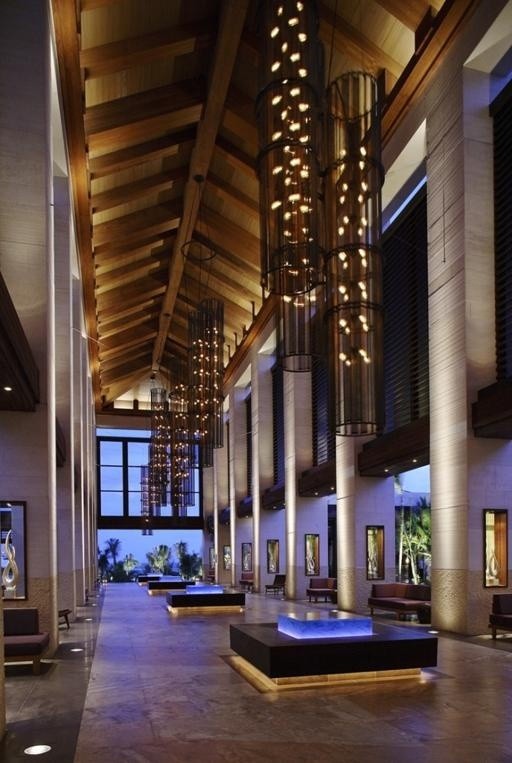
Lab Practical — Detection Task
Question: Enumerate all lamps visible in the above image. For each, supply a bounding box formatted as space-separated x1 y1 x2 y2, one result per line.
276 109 325 373
135 172 230 538
256 0 324 297
324 65 387 439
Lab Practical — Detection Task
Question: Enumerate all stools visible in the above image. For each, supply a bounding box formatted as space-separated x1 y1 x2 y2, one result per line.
58 606 74 630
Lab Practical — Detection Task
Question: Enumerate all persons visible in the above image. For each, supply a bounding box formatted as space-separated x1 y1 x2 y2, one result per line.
98 555 111 583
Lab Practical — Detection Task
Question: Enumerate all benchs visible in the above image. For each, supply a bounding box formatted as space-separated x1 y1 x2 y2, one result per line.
207 569 215 581
2 604 51 678
305 575 337 604
486 590 512 641
366 580 431 621
239 572 254 591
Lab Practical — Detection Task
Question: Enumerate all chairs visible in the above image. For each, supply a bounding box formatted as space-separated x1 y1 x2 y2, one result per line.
263 573 286 596
